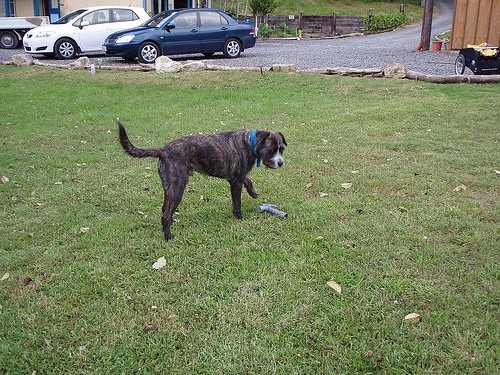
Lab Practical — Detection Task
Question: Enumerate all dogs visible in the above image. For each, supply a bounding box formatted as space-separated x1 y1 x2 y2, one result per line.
113 119 288 243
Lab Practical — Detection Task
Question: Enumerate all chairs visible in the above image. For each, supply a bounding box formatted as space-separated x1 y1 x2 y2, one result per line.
90 12 99 24
176 16 187 26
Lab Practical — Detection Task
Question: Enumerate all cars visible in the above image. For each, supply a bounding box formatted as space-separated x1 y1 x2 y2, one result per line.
22 4 157 61
102 8 258 64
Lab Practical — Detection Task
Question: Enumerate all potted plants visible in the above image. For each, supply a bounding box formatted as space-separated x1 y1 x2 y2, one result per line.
445 37 451 50
432 35 443 51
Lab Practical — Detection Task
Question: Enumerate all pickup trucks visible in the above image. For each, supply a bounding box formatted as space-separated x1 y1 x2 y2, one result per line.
0 16 50 49
